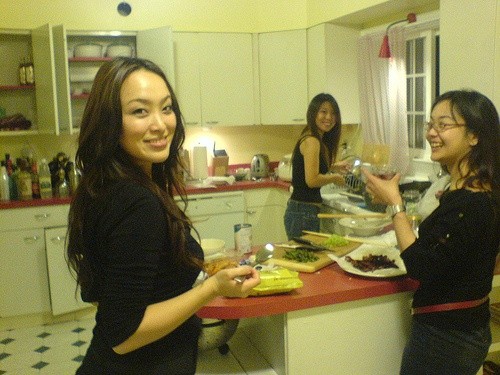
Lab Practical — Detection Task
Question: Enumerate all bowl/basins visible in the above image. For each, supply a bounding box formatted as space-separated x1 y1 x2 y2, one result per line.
73 43 102 57
106 44 133 57
197 238 226 257
338 217 392 236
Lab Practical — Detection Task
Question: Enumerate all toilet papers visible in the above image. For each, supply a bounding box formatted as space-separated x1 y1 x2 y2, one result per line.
192 145 208 179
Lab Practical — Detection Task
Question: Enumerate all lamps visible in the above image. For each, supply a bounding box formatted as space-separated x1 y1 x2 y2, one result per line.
378 13 416 60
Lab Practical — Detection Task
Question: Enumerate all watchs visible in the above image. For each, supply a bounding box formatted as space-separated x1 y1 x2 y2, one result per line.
386 204 406 216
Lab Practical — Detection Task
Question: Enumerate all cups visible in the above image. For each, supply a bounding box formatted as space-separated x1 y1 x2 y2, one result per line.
402 189 419 214
369 164 395 204
234 223 253 254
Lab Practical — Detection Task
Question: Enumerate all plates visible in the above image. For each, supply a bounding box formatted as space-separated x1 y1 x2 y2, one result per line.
340 251 407 278
250 269 304 296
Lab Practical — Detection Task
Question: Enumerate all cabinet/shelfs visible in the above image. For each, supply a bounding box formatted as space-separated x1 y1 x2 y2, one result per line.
0 23 59 136
243 187 276 246
1 198 94 316
170 191 244 257
53 24 176 135
134 31 255 127
256 28 308 126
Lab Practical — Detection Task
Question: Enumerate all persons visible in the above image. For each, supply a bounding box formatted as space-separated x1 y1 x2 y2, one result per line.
64 56 260 375
283 93 352 241
366 90 500 375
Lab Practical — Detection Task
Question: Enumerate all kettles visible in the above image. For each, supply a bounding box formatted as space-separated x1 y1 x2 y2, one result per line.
278 153 293 182
250 153 269 178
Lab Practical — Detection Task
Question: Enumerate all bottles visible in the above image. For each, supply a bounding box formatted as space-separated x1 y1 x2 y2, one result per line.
0 142 79 200
19 55 35 85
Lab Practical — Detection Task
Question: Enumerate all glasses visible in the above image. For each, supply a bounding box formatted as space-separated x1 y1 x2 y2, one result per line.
422 120 469 133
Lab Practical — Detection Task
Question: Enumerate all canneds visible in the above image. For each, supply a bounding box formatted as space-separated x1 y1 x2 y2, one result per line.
234 223 253 254
407 214 422 232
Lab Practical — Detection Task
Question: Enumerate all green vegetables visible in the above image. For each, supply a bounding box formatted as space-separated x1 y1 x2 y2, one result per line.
282 249 319 263
324 232 349 245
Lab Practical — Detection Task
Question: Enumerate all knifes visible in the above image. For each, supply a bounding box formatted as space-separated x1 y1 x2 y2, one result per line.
293 236 336 252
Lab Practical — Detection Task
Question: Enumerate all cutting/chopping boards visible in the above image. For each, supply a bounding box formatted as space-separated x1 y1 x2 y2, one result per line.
265 233 362 272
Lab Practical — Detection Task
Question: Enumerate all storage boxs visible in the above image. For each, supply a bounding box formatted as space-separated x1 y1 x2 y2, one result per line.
212 150 228 176
